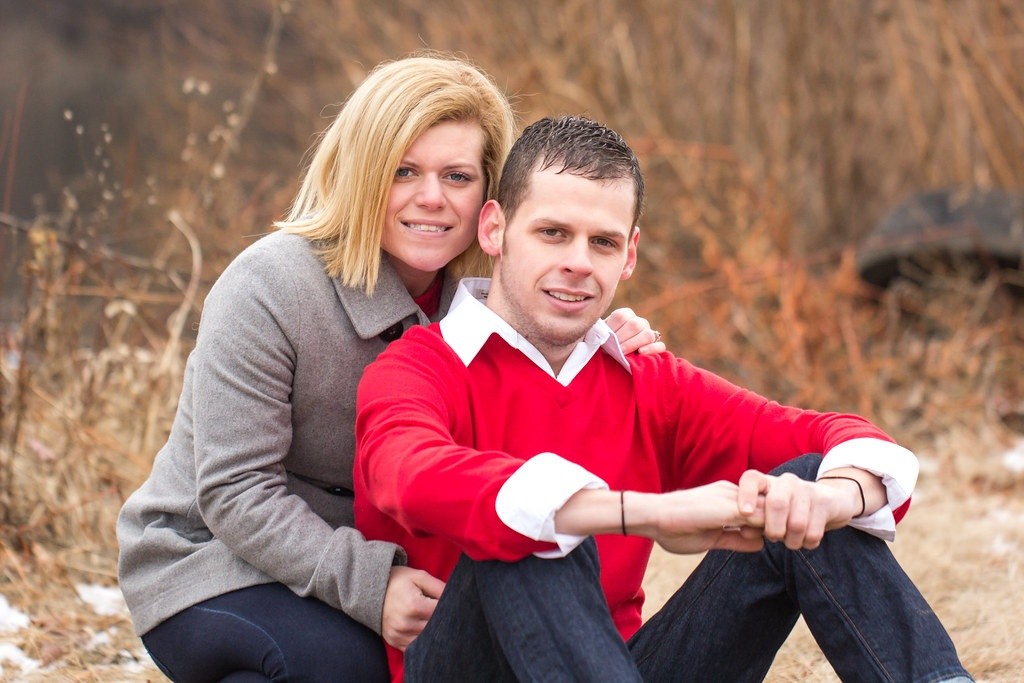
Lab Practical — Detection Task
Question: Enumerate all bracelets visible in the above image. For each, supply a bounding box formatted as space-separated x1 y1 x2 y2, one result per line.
620 492 626 538
817 477 866 521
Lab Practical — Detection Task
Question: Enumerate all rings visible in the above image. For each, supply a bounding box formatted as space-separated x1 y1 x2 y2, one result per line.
652 329 661 342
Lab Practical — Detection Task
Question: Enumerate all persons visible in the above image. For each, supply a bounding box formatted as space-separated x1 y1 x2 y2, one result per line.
121 52 666 683
352 114 977 683
